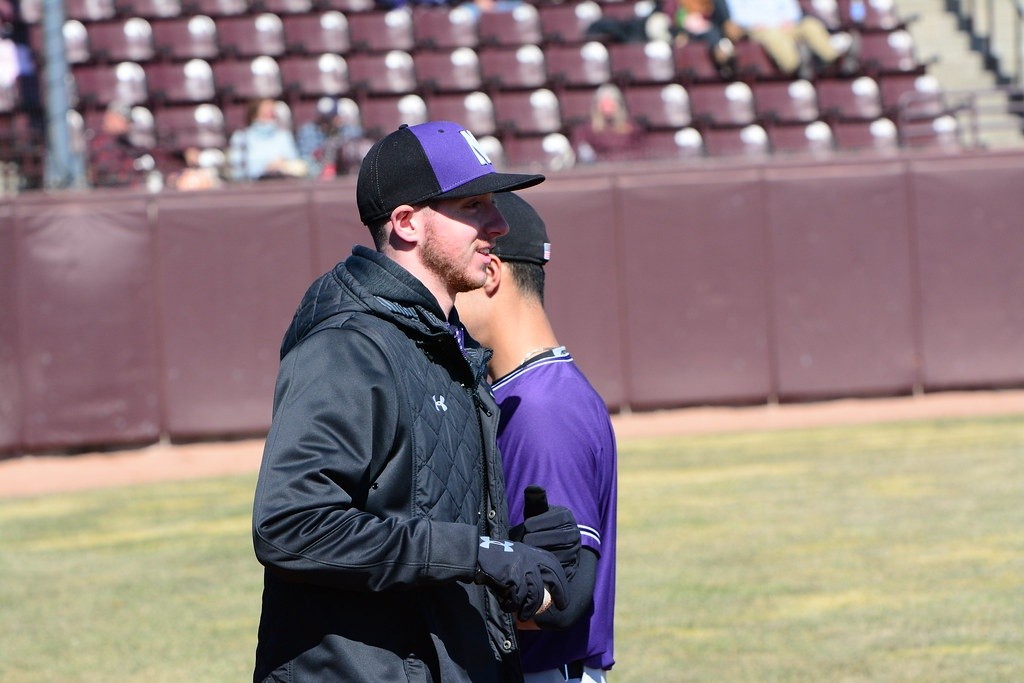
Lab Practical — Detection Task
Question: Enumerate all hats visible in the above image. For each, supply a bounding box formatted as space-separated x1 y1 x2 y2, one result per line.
490 192 551 266
356 120 546 226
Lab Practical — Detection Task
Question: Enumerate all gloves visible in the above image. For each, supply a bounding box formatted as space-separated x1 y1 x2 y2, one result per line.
509 484 581 582
475 536 570 622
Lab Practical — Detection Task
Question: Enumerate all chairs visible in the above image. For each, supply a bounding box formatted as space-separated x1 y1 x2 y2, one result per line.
0 0 974 192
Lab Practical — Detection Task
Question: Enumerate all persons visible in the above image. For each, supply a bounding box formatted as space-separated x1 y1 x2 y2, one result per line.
252 120 582 682
648 0 880 82
572 83 650 165
454 190 615 683
86 98 378 189
